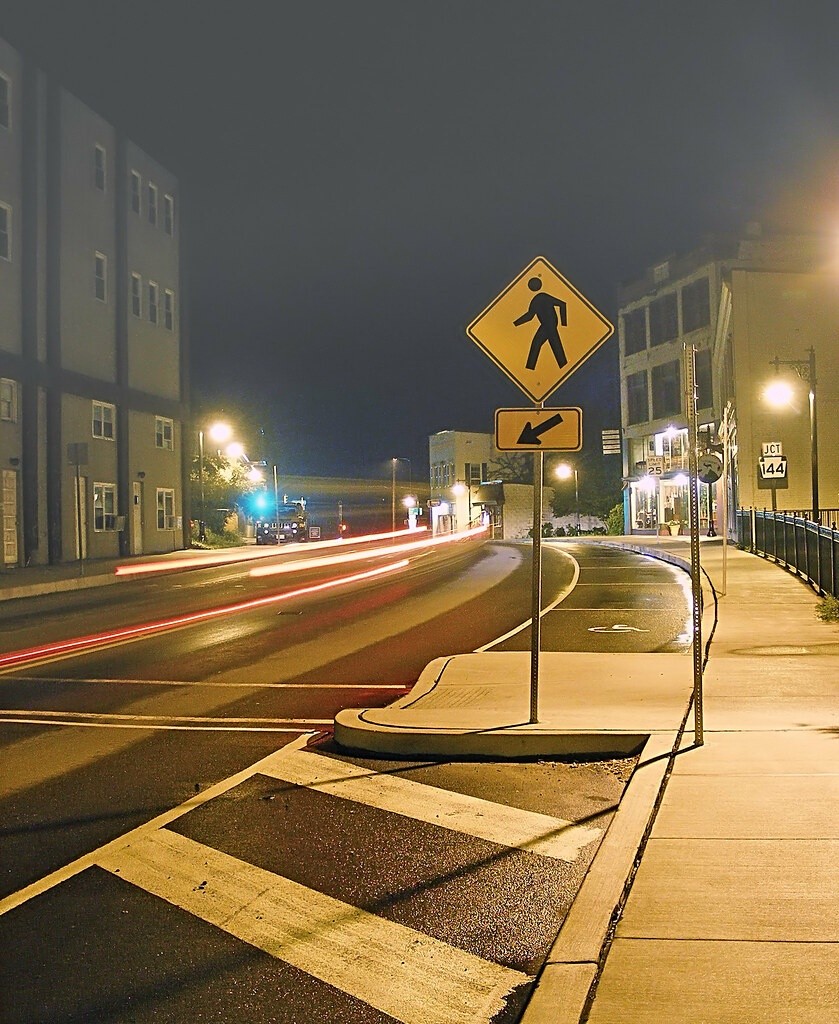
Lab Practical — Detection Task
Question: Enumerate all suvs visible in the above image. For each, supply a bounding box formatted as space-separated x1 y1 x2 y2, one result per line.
256 502 308 545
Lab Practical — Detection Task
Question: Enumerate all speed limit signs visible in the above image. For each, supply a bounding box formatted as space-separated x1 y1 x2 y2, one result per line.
646 456 663 477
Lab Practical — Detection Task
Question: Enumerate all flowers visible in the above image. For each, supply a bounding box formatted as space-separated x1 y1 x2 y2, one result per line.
668 519 688 529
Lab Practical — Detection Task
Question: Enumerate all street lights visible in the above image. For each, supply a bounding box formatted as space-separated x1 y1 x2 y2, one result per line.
453 478 473 529
762 345 820 523
665 425 719 538
555 468 581 536
392 457 412 532
198 422 233 544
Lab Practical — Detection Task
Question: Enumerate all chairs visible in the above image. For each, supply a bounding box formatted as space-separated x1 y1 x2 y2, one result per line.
289 509 295 512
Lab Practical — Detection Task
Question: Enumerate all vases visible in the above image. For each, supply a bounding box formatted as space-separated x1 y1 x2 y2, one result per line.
679 529 688 535
669 525 680 536
